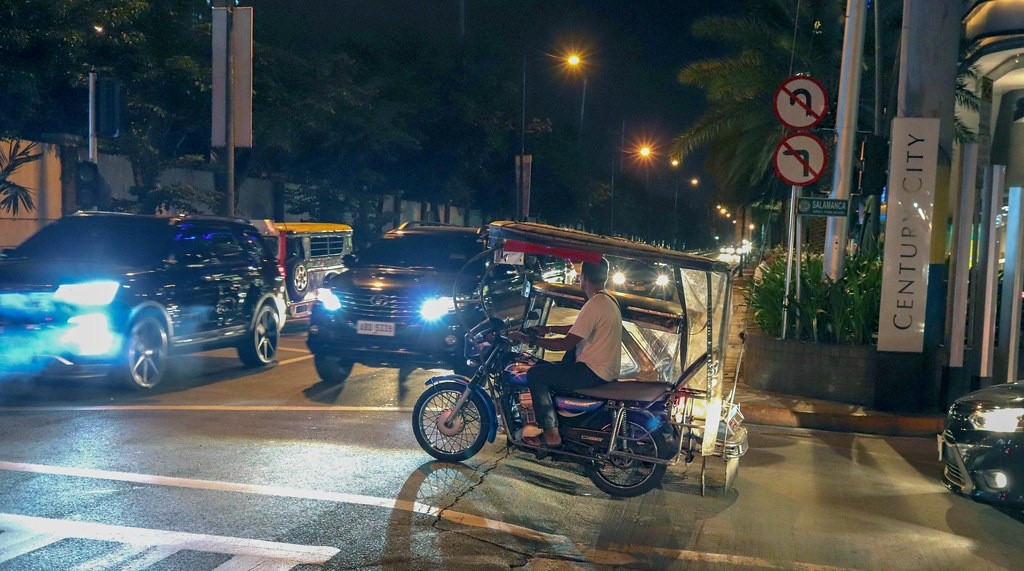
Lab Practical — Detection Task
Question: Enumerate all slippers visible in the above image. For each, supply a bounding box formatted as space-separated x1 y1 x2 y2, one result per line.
521 433 563 450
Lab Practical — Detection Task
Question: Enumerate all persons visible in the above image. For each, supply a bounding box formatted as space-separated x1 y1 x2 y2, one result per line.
506 256 622 450
738 251 778 341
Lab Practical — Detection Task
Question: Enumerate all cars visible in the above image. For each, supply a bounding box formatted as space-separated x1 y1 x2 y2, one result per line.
305 216 486 381
0 211 287 389
939 378 1023 519
539 252 581 284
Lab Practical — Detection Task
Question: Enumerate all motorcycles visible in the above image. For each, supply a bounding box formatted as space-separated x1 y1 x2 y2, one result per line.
412 290 680 497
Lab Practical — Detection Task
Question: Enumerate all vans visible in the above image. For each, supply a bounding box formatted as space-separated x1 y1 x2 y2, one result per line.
253 216 356 330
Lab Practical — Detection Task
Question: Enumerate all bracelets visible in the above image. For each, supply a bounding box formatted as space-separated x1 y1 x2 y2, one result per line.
532 336 539 345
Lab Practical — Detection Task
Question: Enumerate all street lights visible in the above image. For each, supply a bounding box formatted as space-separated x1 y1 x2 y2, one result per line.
520 37 587 227
628 124 651 248
670 154 755 256
